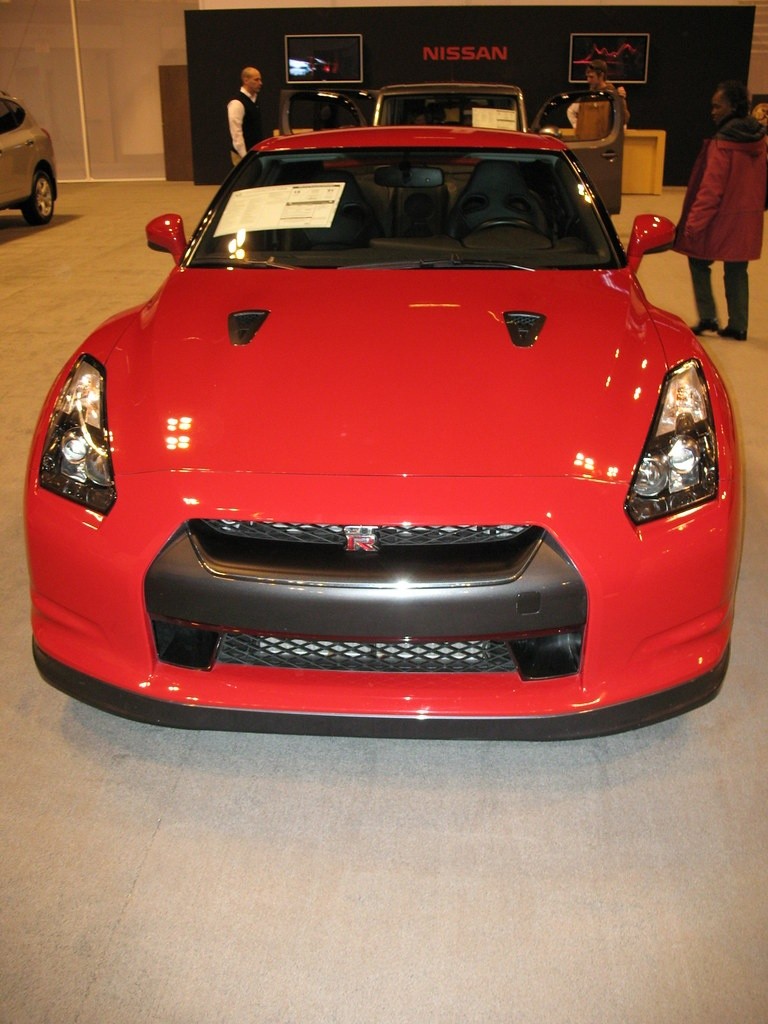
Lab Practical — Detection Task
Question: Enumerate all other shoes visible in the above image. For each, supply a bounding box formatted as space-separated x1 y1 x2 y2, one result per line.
717 327 747 340
690 319 719 335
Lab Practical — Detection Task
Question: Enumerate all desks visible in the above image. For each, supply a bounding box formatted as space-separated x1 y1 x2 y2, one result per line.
560 127 667 196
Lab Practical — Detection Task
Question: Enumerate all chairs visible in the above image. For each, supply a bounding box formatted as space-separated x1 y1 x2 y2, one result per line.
451 159 551 240
300 170 368 250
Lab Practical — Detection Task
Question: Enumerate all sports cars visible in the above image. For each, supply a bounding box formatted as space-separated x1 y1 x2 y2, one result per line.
32 126 750 734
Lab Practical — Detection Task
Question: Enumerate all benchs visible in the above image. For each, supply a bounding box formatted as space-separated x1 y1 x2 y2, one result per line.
353 173 474 237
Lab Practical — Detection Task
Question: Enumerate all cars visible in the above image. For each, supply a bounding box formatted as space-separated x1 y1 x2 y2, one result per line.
281 85 629 226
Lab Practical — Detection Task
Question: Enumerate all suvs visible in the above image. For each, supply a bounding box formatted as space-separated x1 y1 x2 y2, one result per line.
0 91 59 225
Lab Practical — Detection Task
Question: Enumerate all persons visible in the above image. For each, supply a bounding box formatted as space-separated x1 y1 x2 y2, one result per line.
225 66 263 166
673 78 768 341
408 111 432 124
566 60 631 130
